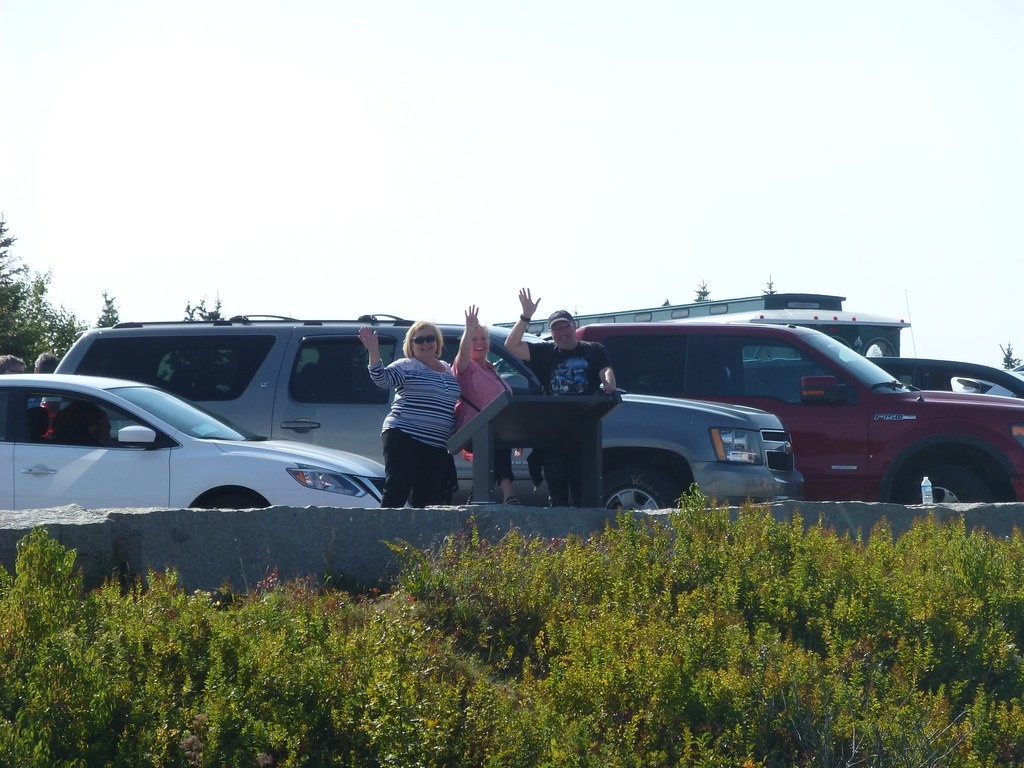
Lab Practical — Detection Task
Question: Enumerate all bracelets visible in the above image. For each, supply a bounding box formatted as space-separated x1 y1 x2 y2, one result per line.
520 314 530 322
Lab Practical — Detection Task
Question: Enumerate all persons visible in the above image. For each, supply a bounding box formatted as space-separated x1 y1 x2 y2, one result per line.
0 352 60 374
358 321 461 509
504 287 616 508
58 404 113 449
450 305 524 506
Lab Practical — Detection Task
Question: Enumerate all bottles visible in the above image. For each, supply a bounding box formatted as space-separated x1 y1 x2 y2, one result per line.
921 476 934 504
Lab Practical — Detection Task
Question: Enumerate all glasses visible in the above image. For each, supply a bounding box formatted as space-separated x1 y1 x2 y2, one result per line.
411 335 438 345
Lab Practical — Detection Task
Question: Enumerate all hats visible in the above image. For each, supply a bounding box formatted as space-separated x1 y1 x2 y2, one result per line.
547 310 575 329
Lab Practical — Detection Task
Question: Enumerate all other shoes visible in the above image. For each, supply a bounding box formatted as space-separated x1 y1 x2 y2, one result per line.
503 496 522 506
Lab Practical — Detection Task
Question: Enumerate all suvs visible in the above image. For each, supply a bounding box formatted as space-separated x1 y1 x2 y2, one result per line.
40 312 806 511
573 322 1024 504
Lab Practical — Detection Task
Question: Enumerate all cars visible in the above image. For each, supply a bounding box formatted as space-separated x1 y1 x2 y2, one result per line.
743 357 1024 400
1 373 411 510
